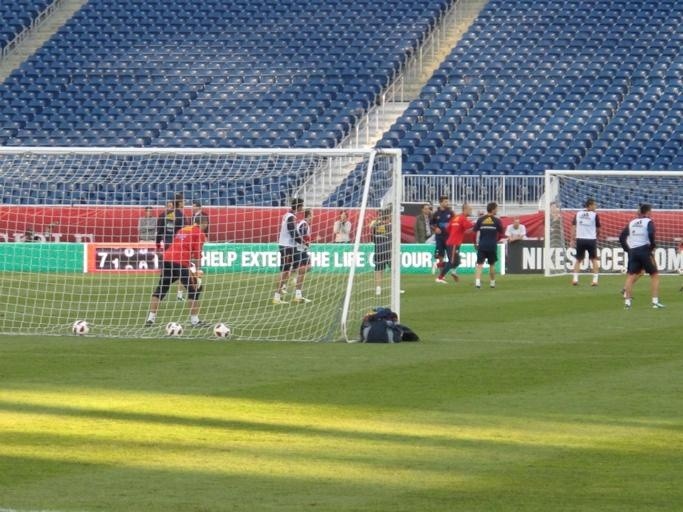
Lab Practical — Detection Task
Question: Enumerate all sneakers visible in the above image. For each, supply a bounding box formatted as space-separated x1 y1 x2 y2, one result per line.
621 288 634 311
650 302 665 309
292 297 311 305
432 262 496 288
591 282 599 286
144 319 154 327
272 298 289 304
177 297 186 302
571 280 579 286
374 286 381 297
192 320 213 328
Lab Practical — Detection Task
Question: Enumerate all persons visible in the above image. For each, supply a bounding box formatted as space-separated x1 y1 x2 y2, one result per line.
570 198 601 286
544 202 564 248
505 218 529 242
333 211 351 243
281 209 314 293
620 214 654 299
620 204 665 309
272 198 312 305
138 196 212 329
370 203 503 296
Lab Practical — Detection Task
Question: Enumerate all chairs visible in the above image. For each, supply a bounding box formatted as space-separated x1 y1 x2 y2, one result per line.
0 0 682 208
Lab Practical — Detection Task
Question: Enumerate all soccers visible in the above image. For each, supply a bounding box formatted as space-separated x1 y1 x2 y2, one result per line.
71 320 89 335
165 322 182 336
213 322 230 338
